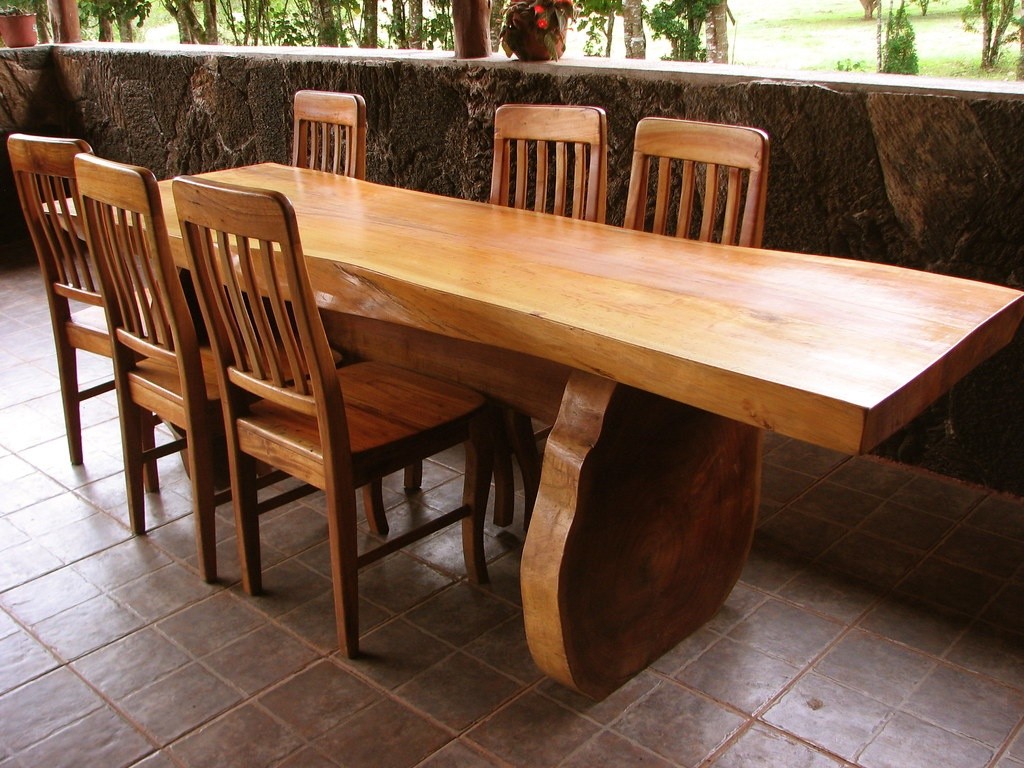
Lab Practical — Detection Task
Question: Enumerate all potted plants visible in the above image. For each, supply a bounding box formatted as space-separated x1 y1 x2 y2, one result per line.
0 0 53 48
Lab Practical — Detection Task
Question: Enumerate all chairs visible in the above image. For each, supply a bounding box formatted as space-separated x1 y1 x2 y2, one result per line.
505 116 769 532
7 134 498 663
403 103 608 528
291 90 367 181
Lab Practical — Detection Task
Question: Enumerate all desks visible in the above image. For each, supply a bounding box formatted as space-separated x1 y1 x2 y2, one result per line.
42 160 1024 703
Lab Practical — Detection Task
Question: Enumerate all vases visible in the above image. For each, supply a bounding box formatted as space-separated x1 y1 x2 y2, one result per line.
512 13 563 61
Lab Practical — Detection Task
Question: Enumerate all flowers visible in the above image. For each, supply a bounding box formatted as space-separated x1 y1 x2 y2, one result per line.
498 0 578 62
